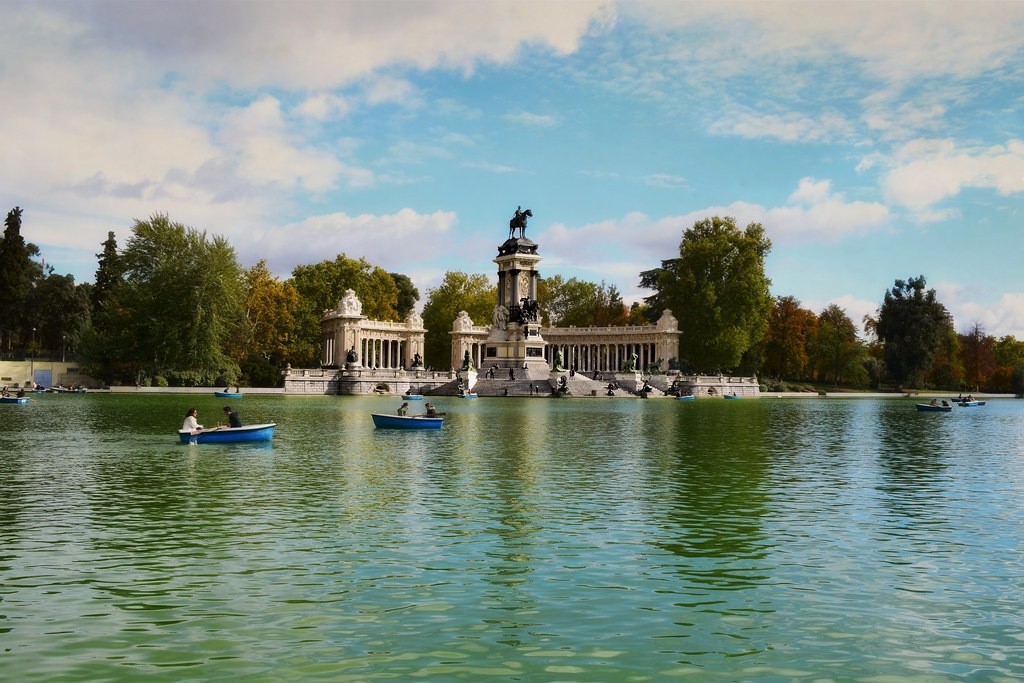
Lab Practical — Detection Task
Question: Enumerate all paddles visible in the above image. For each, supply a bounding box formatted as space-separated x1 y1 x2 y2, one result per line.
191 425 230 436
409 412 446 417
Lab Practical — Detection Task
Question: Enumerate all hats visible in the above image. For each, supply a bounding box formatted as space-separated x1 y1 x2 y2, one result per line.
404 402 408 406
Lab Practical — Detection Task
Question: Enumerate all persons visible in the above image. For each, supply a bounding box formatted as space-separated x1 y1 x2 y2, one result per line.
223 406 242 428
929 392 975 407
182 406 205 431
719 371 723 382
2 385 25 398
513 206 523 227
348 346 358 361
413 353 422 367
405 389 414 395
557 364 682 397
397 402 410 416
224 385 239 393
514 296 541 325
425 403 437 418
458 362 539 396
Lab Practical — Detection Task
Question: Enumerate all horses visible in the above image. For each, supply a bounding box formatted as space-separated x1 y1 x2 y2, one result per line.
509 210 533 239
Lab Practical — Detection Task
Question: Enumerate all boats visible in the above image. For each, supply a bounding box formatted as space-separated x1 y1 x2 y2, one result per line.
214 391 243 398
456 393 478 398
0 395 32 405
371 412 444 429
32 387 88 394
401 394 424 400
951 397 986 407
675 394 695 401
724 394 740 400
177 422 276 444
915 403 953 412
